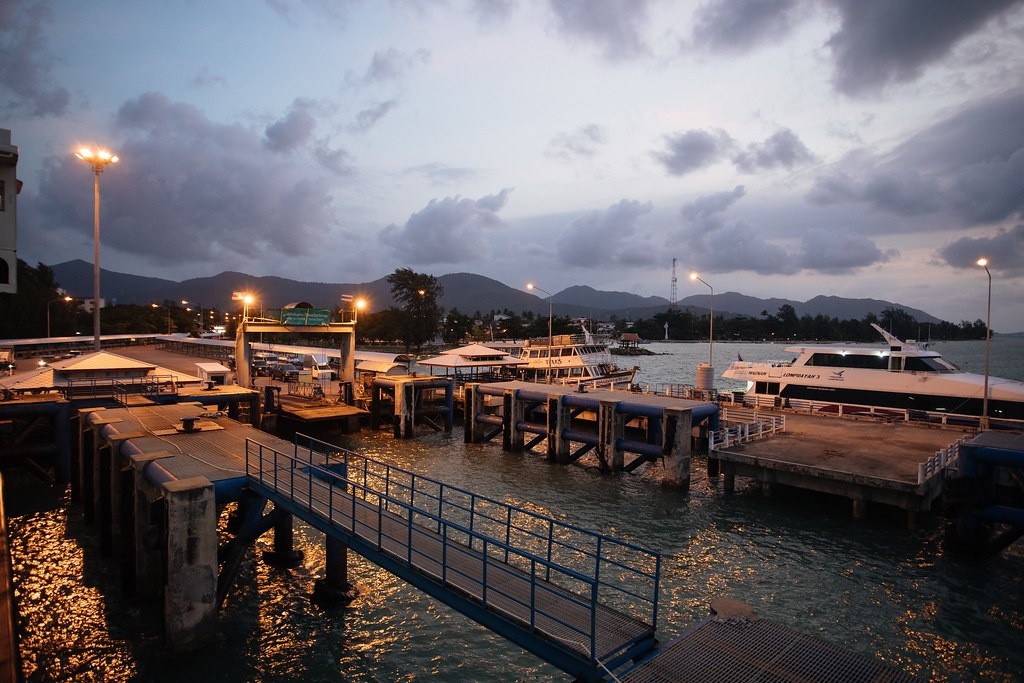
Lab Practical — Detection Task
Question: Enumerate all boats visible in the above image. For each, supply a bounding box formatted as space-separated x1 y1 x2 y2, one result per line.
719 322 1024 423
492 333 638 390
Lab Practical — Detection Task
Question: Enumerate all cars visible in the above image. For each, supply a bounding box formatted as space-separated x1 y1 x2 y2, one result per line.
40 350 81 368
270 362 299 381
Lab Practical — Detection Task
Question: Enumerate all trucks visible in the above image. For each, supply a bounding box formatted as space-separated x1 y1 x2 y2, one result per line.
304 362 337 380
252 354 272 376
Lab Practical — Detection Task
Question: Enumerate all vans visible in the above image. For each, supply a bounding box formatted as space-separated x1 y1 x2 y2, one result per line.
279 356 301 369
256 351 278 367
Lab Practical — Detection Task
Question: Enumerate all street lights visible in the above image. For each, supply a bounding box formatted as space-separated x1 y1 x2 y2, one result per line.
182 301 203 334
357 300 366 314
244 295 251 321
47 298 73 351
76 147 118 351
151 304 170 334
527 283 551 383
418 289 429 346
977 259 992 426
689 273 713 366
